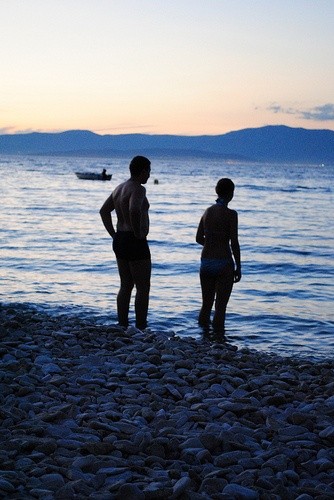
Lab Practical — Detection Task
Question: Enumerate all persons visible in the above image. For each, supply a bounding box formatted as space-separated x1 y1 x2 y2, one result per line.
102 169 106 175
100 155 151 328
196 178 241 332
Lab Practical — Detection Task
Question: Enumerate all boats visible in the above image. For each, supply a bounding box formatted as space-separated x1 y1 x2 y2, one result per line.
74 171 113 181
